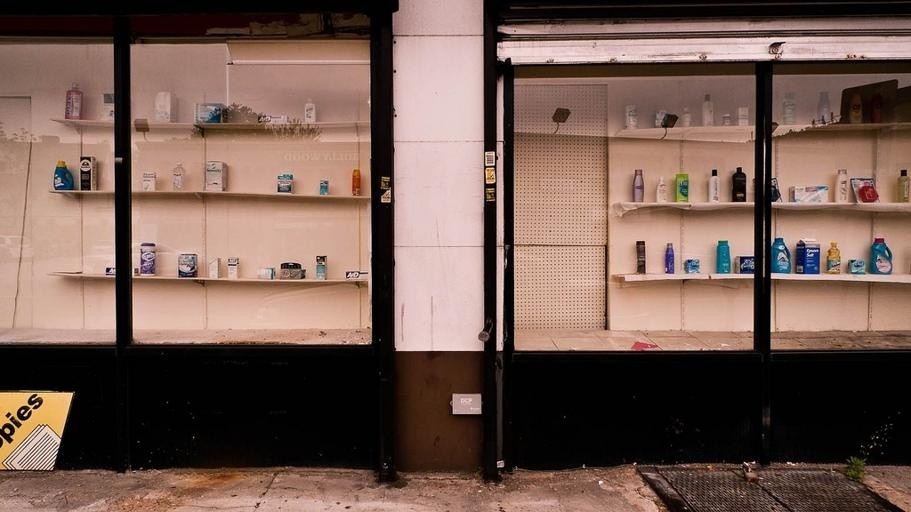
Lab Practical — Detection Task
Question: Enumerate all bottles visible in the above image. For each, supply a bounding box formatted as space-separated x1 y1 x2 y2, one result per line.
63 80 84 121
316 255 327 281
634 240 646 274
631 168 645 202
835 170 850 203
170 157 185 193
781 91 797 126
177 253 199 278
707 167 722 203
352 168 361 197
656 175 668 204
701 87 714 127
895 168 911 205
673 159 690 203
304 97 316 123
138 242 156 276
722 114 731 126
664 242 674 274
715 239 732 274
681 105 692 128
815 91 832 125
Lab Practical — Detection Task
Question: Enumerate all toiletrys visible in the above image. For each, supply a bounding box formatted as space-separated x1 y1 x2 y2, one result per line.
815 90 831 123
708 169 721 201
655 176 668 202
633 167 646 201
783 91 797 124
676 173 688 202
701 93 715 126
665 243 676 273
717 239 731 273
896 169 910 202
351 169 361 195
302 98 316 125
681 105 691 126
834 169 849 202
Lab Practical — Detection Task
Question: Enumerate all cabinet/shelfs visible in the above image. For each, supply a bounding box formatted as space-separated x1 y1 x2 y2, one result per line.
47 117 371 285
611 122 911 287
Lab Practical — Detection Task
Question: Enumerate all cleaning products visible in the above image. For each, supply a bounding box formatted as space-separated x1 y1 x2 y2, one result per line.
65 81 84 119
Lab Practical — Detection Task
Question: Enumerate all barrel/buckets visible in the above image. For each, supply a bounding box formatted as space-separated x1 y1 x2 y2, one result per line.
54 159 74 189
870 236 894 275
772 238 792 272
